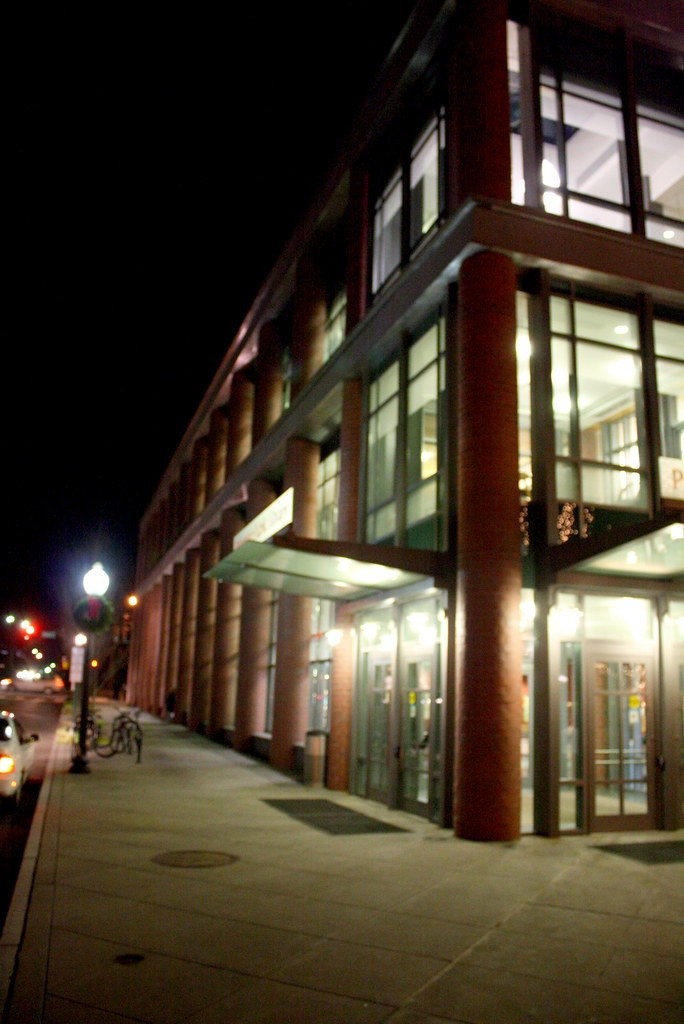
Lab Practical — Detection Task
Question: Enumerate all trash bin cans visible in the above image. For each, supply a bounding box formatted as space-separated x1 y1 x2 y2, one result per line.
302 729 327 788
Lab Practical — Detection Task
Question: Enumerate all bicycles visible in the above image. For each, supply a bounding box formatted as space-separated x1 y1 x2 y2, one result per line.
71 704 142 764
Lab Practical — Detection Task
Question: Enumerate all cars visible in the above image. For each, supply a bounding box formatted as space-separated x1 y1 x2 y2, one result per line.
13 670 65 695
0 711 40 809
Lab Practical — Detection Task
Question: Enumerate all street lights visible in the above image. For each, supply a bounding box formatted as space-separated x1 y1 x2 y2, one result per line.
66 560 111 775
